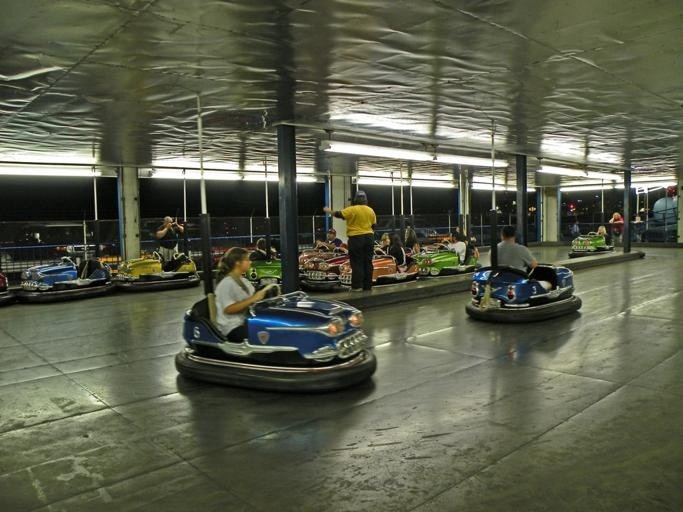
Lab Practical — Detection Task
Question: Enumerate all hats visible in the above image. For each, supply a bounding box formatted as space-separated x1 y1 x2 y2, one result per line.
327 228 336 234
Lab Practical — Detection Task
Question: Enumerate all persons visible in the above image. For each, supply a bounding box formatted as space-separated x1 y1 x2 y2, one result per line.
630 216 645 242
608 212 624 243
247 228 466 275
596 225 612 246
214 247 280 344
155 216 184 261
570 220 579 237
323 190 377 292
0 250 11 262
488 225 552 291
